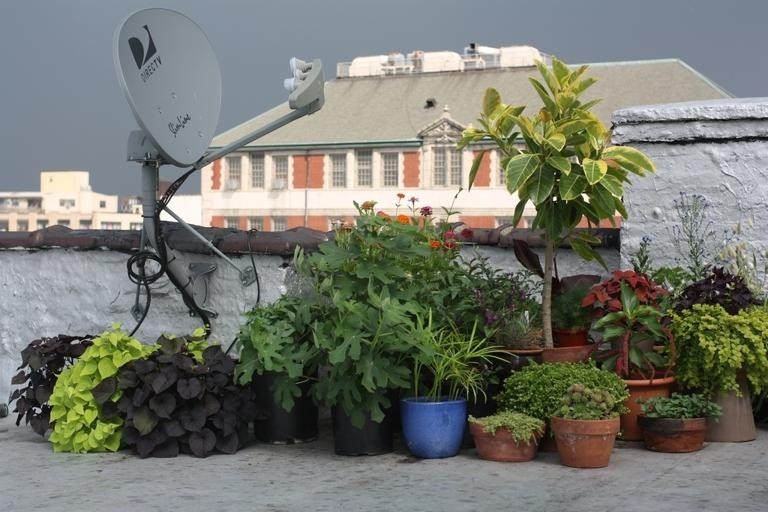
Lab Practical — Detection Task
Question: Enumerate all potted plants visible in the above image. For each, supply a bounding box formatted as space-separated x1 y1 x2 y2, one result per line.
468 410 546 463
672 300 768 445
541 379 623 467
580 267 676 443
397 307 517 463
551 286 591 347
231 293 323 448
634 388 724 455
314 304 412 459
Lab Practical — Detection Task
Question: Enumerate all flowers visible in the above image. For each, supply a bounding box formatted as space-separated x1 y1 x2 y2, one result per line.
359 188 532 326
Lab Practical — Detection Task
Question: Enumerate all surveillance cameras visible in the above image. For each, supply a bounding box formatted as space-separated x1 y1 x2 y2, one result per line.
470 43 479 49
